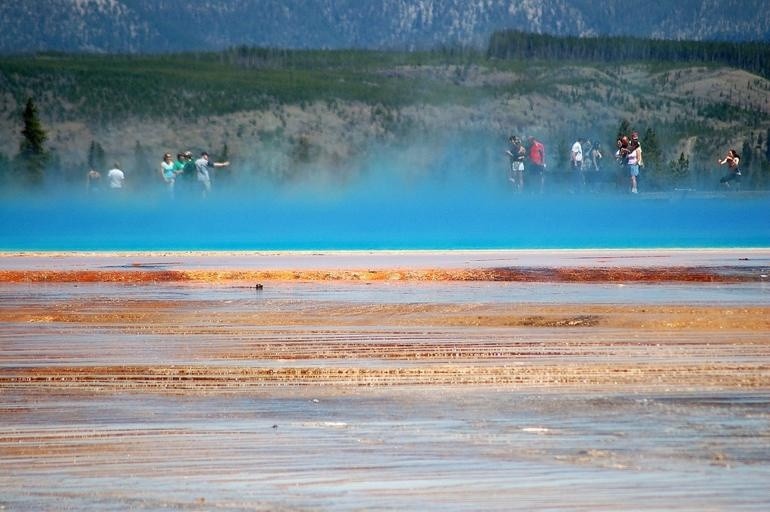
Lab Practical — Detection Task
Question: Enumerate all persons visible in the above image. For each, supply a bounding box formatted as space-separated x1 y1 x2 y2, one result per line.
501 132 548 198
613 131 645 195
564 133 605 199
85 164 102 196
158 149 232 196
107 160 126 195
717 149 742 192
